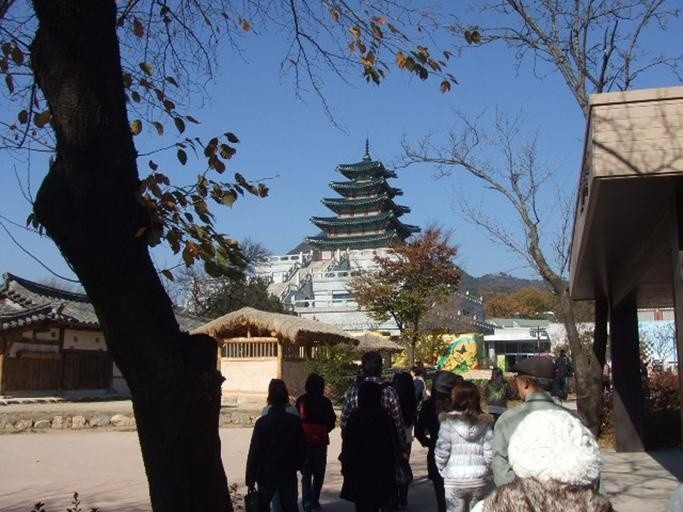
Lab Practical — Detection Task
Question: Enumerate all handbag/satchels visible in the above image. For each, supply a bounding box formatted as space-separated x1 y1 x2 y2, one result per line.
244 486 270 512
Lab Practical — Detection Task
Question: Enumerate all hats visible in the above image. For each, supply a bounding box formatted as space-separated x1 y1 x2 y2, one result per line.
510 358 556 380
506 408 602 488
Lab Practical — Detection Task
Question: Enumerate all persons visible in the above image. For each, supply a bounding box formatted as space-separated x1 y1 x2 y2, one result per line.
640 351 653 379
237 348 614 510
